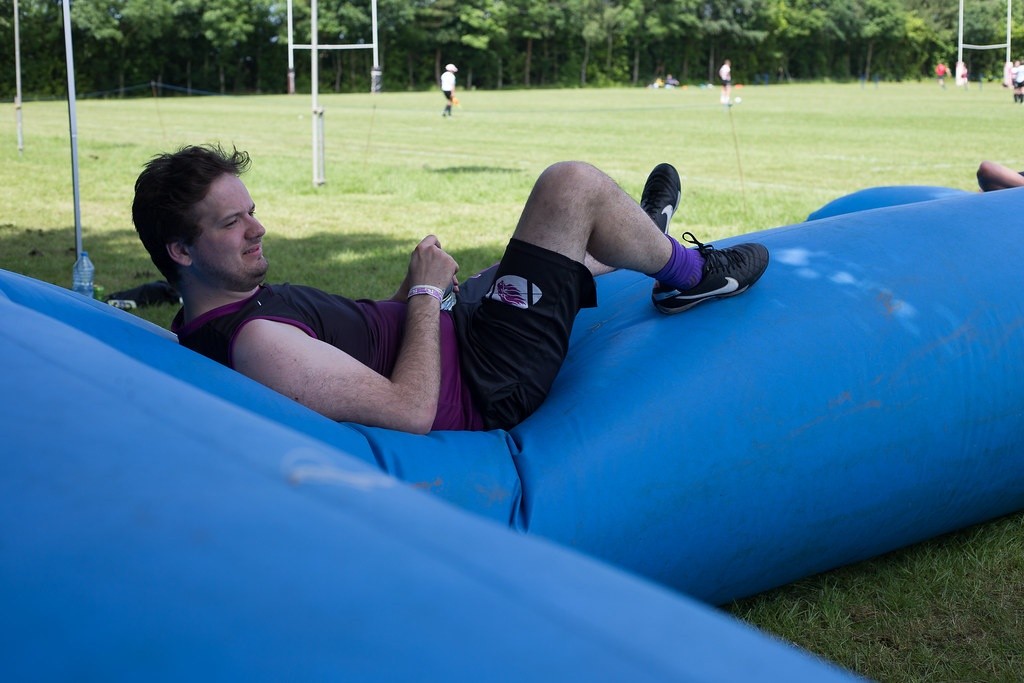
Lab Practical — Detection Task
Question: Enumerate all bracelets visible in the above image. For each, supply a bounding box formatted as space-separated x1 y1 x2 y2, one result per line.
407 284 444 304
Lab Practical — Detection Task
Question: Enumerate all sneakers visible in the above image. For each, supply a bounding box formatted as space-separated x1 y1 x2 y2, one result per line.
640 163 681 234
652 232 769 314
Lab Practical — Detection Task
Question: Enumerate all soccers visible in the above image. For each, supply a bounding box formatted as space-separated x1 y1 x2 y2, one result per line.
735 96 742 104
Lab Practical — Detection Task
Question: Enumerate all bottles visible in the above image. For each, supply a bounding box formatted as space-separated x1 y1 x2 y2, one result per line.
72 251 94 299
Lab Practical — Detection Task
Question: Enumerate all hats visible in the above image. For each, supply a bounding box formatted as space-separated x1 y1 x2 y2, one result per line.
446 64 458 72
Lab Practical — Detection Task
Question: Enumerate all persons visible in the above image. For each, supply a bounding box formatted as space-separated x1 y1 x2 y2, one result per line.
976 160 1024 192
719 60 732 105
132 146 768 434
441 63 458 118
662 73 679 86
935 60 968 85
1010 60 1024 103
